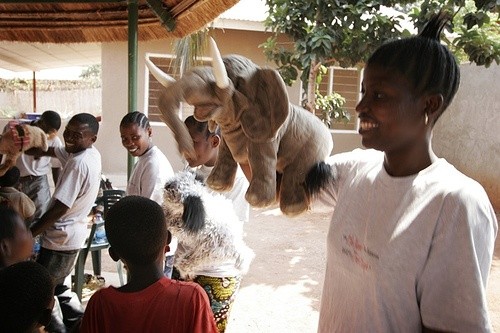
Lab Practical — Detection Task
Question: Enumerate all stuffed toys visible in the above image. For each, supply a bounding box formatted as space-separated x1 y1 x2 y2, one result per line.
160 173 250 282
144 36 334 214
1 122 48 174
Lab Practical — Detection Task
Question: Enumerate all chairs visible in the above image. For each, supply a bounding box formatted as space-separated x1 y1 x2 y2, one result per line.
75 190 128 303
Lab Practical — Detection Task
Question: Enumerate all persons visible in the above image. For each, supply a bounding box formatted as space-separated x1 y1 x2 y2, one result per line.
238 3 498 333
32 110 64 185
80 194 219 333
0 120 52 228
171 114 256 333
0 206 34 271
0 112 101 333
75 174 117 284
0 163 38 241
0 260 57 333
120 111 179 279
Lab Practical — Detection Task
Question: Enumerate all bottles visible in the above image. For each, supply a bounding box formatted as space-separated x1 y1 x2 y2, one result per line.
32 234 42 264
95 212 109 244
